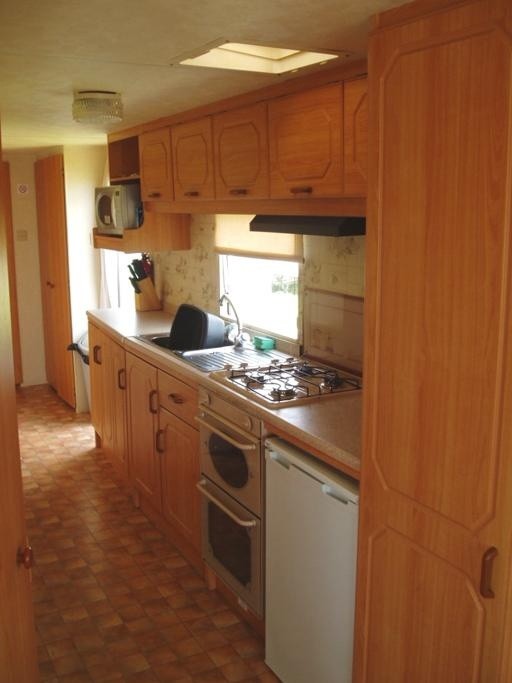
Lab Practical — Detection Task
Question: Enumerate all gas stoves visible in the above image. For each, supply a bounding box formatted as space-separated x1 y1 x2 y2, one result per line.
210 355 363 410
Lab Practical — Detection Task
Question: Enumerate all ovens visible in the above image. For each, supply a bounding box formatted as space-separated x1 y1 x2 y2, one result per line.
195 379 265 621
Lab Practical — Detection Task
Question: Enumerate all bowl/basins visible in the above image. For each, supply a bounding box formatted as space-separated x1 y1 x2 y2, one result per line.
167 300 226 352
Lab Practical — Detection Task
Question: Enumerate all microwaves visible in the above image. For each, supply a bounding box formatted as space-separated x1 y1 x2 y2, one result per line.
92 184 145 236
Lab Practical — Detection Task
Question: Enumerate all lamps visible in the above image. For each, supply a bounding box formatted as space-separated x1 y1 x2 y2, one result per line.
72 91 124 128
166 36 353 76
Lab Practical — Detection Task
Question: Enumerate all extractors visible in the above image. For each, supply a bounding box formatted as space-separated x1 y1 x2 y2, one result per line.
249 215 367 236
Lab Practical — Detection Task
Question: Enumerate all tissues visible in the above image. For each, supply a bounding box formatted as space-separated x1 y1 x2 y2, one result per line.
251 335 275 350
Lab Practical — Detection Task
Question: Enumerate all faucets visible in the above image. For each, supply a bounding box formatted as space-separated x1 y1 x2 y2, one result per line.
215 293 246 348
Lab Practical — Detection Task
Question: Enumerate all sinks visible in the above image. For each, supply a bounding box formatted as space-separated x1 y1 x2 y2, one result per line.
133 331 237 352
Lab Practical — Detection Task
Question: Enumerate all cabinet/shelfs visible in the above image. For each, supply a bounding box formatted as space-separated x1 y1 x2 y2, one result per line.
138 102 213 214
353 0 511 682
344 59 369 218
32 153 121 413
127 337 201 582
212 65 345 217
88 315 126 483
93 123 191 254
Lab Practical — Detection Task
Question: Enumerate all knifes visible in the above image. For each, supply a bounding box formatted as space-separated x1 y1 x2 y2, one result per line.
126 258 145 294
